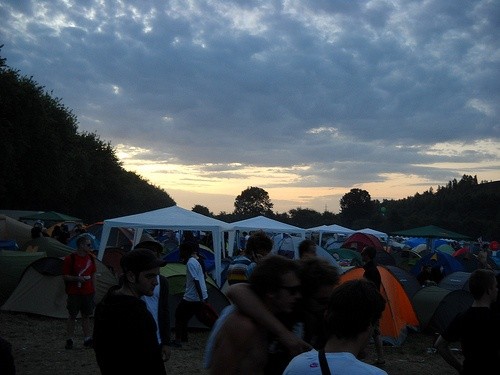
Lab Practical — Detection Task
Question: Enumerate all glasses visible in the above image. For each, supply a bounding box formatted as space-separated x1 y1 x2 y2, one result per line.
84 243 90 246
278 285 301 295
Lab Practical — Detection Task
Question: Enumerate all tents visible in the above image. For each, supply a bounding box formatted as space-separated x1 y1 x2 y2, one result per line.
0 196 500 350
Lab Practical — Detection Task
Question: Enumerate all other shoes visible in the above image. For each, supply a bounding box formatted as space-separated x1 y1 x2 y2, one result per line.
356 352 368 360
171 334 188 343
370 360 385 366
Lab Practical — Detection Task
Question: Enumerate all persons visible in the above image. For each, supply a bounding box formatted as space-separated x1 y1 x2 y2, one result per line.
206 255 390 375
59 233 99 347
182 231 199 257
341 247 387 366
433 270 500 375
172 239 223 348
94 248 168 375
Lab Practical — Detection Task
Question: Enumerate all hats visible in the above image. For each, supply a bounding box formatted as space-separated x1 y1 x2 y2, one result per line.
121 248 167 273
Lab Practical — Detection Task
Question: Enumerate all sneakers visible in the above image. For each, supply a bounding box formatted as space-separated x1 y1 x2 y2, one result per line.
65 338 73 349
84 338 95 349
